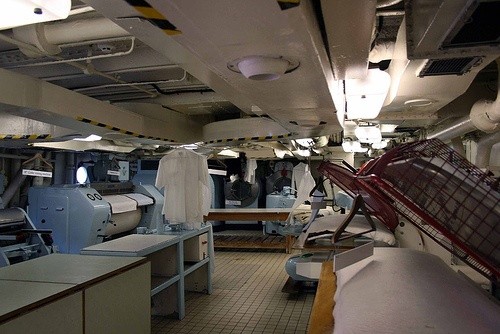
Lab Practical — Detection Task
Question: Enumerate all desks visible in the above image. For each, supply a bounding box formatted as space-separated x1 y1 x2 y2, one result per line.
0 252 150 334
203 208 297 255
81 219 214 321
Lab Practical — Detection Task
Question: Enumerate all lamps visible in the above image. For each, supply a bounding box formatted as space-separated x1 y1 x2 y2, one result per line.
0 0 391 158
